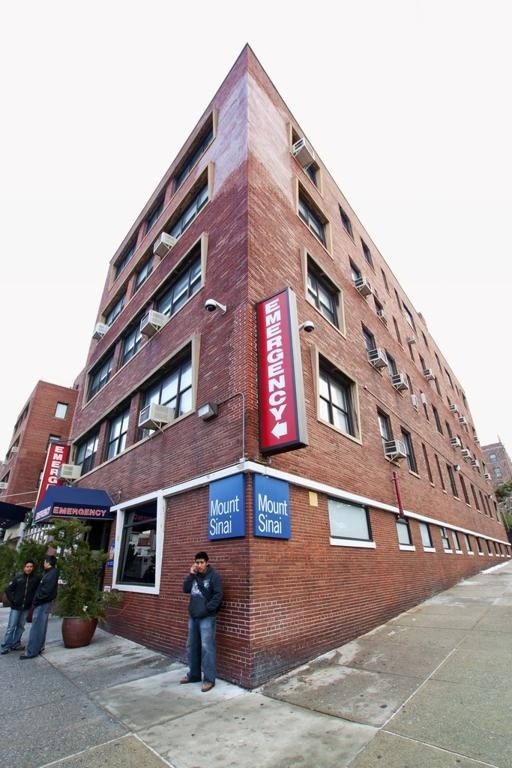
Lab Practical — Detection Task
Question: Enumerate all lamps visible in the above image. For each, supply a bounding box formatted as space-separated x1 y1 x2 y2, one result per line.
197 402 220 421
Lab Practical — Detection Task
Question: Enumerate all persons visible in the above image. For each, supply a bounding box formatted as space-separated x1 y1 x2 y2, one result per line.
20 556 60 659
180 552 224 692
1 559 40 654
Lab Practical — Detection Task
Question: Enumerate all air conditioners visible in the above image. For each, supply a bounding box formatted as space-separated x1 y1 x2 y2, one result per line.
383 437 407 459
140 309 169 337
424 367 491 484
57 464 82 480
152 232 178 258
92 323 108 340
356 275 393 325
293 139 317 165
138 401 174 430
368 347 408 392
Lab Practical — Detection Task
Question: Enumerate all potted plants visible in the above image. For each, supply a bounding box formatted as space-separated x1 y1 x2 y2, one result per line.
57 519 124 649
0 536 49 622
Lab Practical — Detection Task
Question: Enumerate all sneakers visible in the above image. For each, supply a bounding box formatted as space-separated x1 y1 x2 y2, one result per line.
180 676 214 692
0 644 46 660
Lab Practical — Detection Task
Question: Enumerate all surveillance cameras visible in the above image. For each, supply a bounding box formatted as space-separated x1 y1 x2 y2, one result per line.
204 298 218 312
303 321 315 332
